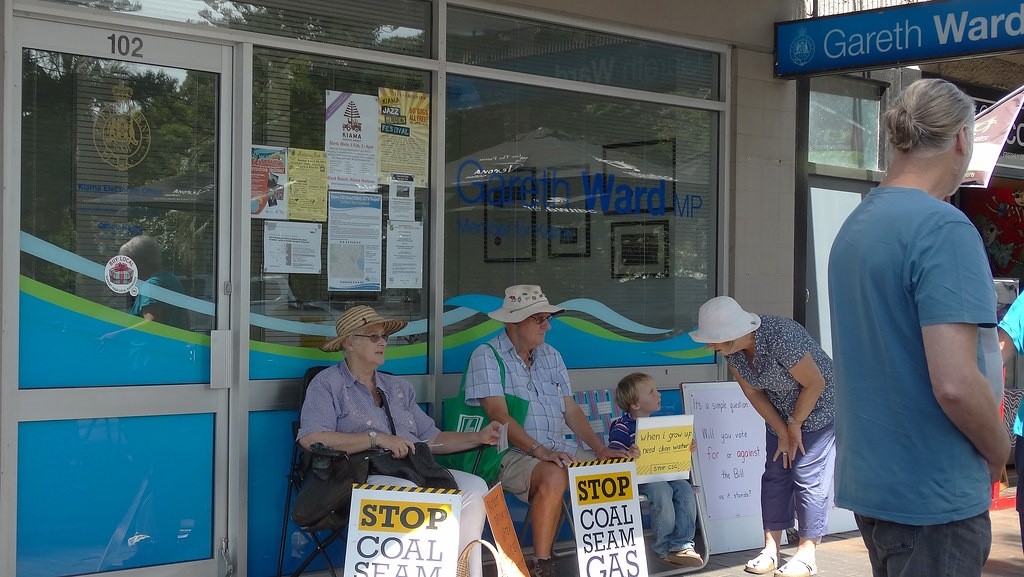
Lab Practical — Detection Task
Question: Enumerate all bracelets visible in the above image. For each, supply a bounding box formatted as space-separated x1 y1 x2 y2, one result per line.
788 416 803 424
529 442 543 455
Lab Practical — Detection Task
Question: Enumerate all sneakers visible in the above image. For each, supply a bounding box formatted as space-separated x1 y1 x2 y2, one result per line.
530 554 556 577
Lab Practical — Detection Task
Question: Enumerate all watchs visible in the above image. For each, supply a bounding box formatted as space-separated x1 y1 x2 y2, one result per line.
368 431 377 448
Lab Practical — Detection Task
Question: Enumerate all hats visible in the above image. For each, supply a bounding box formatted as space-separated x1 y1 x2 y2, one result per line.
687 296 762 344
319 305 408 353
487 285 566 323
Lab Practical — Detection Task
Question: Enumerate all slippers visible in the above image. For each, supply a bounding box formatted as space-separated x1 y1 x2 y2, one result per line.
774 553 817 577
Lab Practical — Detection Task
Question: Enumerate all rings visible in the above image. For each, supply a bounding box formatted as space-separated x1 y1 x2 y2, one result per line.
783 453 787 454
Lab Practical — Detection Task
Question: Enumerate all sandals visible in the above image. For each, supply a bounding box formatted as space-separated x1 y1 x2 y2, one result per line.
655 549 703 568
745 549 783 573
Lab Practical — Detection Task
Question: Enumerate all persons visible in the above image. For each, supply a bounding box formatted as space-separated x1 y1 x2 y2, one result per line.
828 79 1011 577
608 373 703 567
998 290 1024 555
687 296 836 577
465 284 632 577
118 235 190 331
296 304 504 577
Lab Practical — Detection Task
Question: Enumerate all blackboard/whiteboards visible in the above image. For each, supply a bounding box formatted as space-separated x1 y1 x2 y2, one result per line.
680 382 862 553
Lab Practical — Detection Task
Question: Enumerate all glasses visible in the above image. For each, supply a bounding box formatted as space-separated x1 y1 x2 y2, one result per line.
346 334 389 343
530 316 553 326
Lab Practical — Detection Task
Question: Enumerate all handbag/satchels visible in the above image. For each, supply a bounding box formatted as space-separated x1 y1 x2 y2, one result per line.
434 343 528 484
326 451 379 509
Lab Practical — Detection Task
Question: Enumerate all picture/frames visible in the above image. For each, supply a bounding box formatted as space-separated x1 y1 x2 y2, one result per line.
611 220 669 278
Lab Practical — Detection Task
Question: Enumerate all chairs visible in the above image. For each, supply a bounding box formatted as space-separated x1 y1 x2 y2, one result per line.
517 388 709 577
277 367 497 577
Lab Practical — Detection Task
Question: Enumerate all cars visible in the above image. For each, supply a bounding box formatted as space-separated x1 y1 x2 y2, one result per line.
176 275 420 343
